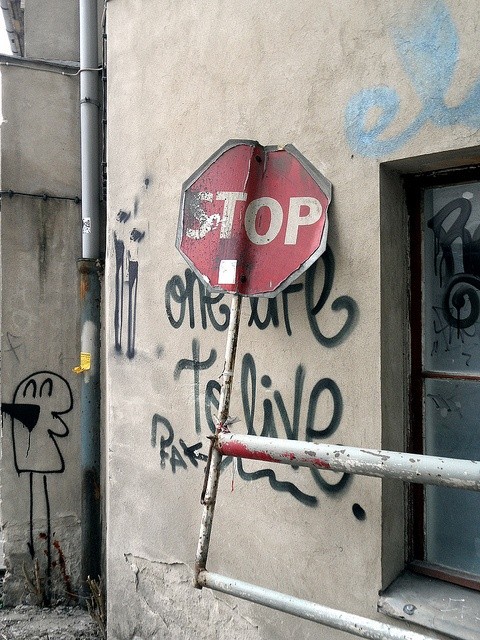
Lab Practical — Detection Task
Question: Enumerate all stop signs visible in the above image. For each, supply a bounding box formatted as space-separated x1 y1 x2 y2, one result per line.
175 137 335 300
